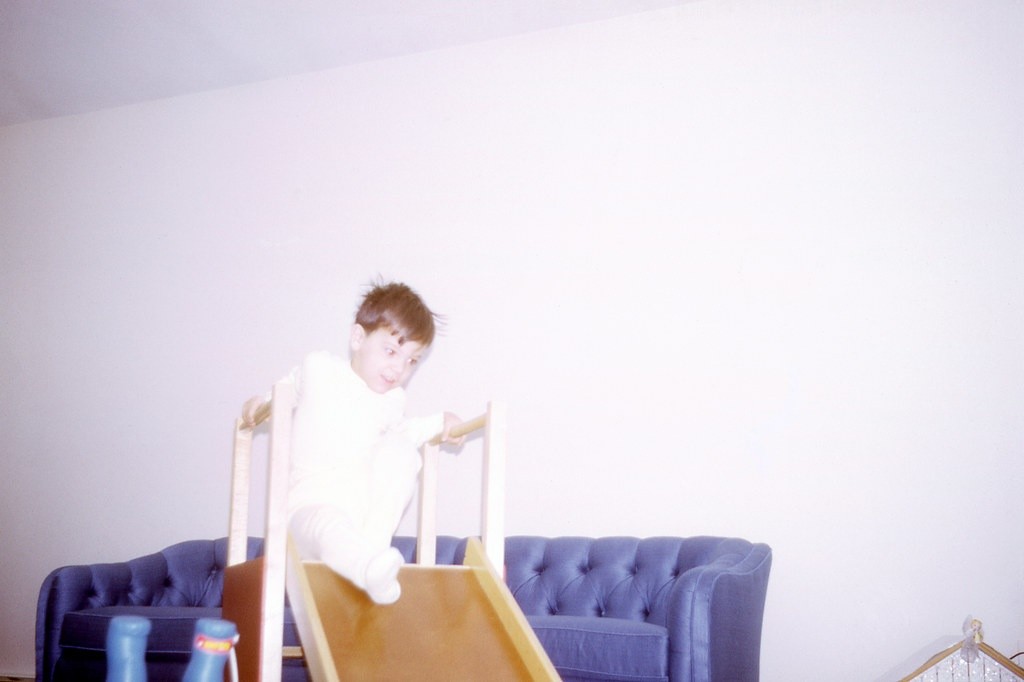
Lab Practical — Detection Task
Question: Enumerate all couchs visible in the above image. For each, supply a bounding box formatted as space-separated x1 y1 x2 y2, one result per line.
34 534 774 682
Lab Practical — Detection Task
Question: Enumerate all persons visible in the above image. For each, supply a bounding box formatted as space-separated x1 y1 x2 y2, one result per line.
241 283 466 605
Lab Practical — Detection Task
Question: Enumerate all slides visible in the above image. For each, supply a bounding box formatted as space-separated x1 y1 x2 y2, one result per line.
284 536 563 682
